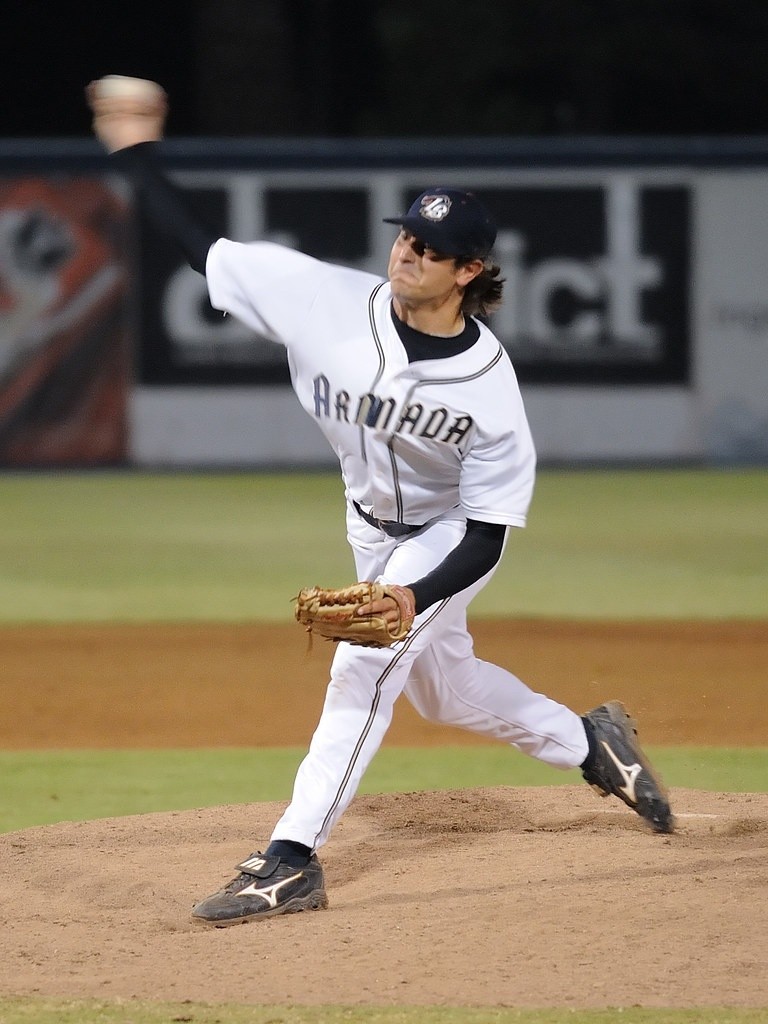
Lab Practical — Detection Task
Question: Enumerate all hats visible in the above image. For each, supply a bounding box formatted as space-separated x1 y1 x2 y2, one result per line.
382 185 498 262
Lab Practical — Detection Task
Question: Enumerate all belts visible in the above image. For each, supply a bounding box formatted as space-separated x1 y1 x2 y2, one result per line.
353 498 429 537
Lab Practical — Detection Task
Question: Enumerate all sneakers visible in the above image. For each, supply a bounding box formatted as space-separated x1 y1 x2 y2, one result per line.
189 842 329 929
579 699 679 835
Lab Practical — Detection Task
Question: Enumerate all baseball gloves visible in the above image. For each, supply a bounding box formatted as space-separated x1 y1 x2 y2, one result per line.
295 582 420 650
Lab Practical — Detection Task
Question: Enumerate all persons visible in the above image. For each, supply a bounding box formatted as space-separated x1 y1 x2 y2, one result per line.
91 74 676 929
